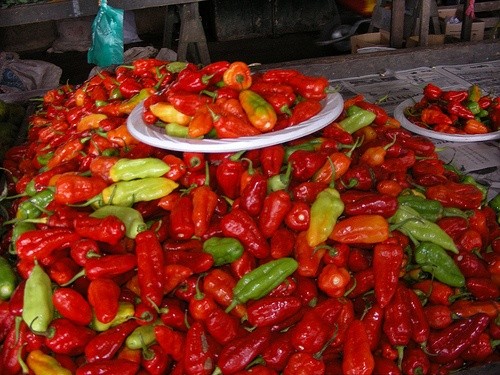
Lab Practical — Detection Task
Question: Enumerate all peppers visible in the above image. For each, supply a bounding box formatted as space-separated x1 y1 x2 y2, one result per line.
0 58 499 375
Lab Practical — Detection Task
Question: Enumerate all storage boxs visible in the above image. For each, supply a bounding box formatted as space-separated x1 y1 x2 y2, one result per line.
351 32 390 54
438 8 485 42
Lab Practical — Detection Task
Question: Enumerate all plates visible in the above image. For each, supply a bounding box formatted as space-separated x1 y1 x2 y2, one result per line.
126 85 344 153
394 94 500 142
